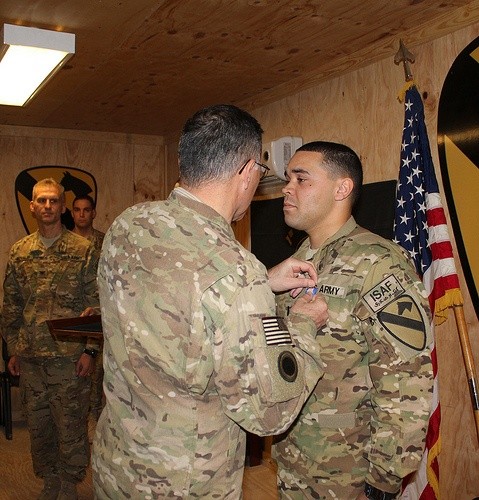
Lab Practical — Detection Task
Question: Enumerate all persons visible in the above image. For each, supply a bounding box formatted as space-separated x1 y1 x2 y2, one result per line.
73 194 105 444
274 141 434 500
0 178 105 500
92 104 329 500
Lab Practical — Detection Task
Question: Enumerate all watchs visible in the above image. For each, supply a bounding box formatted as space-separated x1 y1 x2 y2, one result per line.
83 348 98 358
364 484 394 500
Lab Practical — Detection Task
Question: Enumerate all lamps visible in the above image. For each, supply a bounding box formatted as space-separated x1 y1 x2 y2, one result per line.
0 23 76 108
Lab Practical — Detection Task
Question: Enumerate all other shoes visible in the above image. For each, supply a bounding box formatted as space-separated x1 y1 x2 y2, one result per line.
56 480 78 500
88 418 98 443
37 478 61 500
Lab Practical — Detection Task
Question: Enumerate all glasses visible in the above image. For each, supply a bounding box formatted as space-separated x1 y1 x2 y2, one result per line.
239 159 270 181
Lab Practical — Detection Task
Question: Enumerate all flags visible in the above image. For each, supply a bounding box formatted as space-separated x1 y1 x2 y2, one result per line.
392 79 464 500
